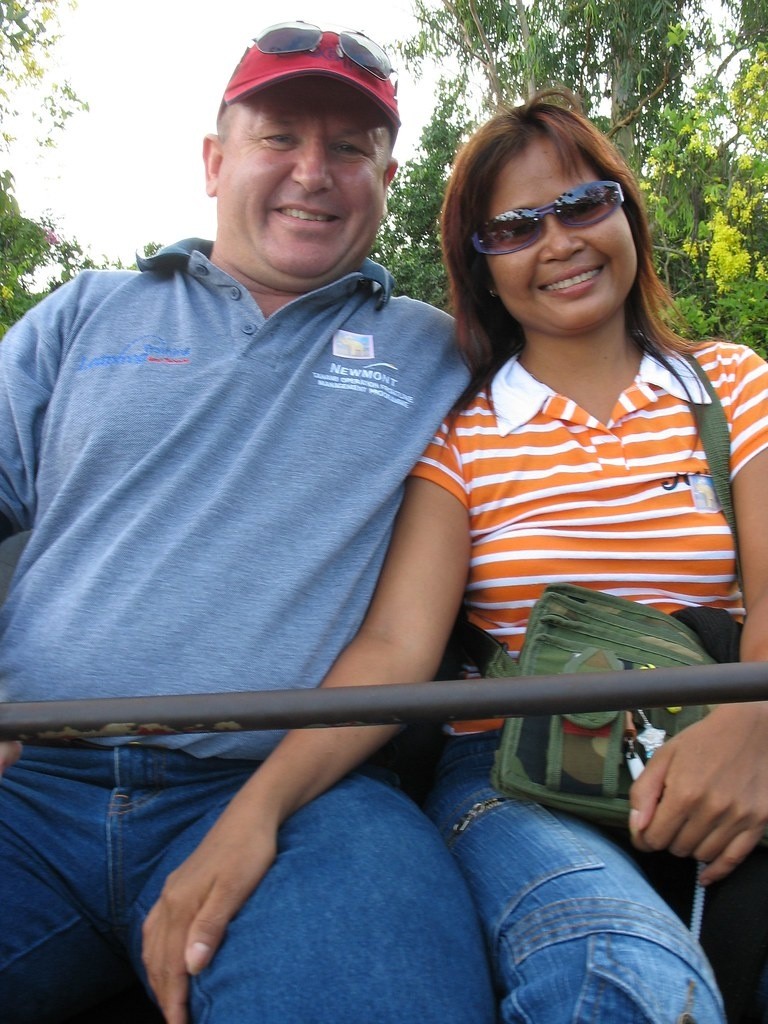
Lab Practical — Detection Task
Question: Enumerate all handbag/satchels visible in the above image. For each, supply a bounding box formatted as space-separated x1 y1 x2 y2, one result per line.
489 579 722 829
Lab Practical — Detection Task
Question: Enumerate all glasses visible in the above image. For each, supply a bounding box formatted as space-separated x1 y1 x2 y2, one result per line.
217 19 399 116
469 180 625 256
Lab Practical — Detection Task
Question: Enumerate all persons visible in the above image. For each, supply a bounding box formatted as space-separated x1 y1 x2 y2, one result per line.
141 96 768 1024
0 22 497 1024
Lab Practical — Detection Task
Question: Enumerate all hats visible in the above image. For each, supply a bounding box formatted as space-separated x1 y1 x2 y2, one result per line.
215 27 403 148
